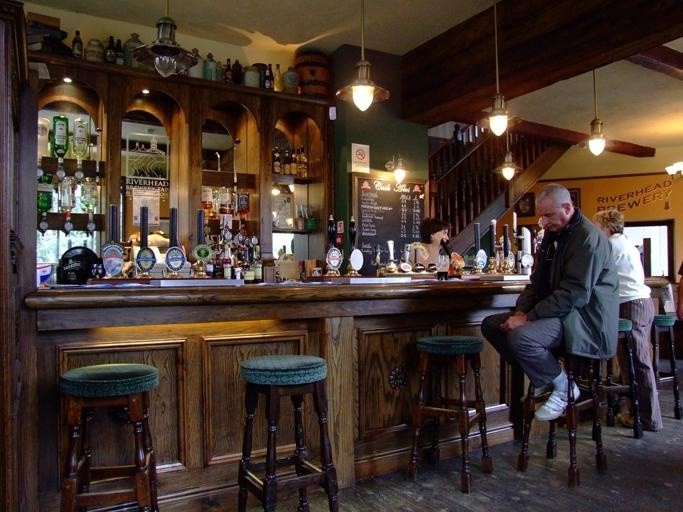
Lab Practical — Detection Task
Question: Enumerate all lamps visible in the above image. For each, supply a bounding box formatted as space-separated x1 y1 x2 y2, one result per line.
485 1 509 137
382 149 407 185
666 159 683 182
130 1 198 79
587 62 608 157
335 0 392 113
493 119 522 182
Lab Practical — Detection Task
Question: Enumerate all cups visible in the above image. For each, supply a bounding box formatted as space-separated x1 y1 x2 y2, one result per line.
435 254 449 280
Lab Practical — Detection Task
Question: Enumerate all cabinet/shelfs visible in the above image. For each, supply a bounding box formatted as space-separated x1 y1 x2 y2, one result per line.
28 51 338 271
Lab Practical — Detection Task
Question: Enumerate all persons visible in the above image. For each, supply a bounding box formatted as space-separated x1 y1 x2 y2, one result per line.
589 209 664 433
479 183 622 421
674 261 683 323
399 216 450 271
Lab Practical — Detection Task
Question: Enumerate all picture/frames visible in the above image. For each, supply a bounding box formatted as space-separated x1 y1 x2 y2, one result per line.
513 192 536 217
568 188 581 209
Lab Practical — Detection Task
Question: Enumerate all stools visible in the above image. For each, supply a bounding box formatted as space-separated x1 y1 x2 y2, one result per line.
600 318 642 440
238 355 338 511
517 350 606 488
56 361 159 512
652 314 682 420
410 335 494 494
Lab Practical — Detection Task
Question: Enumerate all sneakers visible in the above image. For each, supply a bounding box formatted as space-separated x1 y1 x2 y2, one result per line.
534 383 580 421
529 383 553 397
616 411 635 427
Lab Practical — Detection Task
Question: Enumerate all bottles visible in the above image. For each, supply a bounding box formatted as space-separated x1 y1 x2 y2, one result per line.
80 176 97 225
59 176 76 221
291 145 309 177
71 30 123 65
201 186 212 217
72 118 88 170
235 190 249 222
216 187 233 217
51 115 68 167
282 150 290 175
37 118 50 165
214 244 263 283
272 146 281 176
263 62 300 95
35 174 51 221
187 46 245 78
